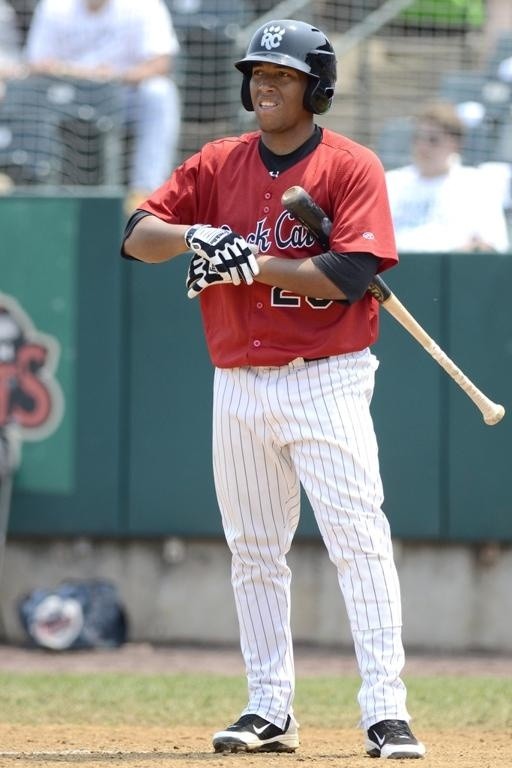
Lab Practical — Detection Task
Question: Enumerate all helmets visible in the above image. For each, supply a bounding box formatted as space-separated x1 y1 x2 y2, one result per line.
232 18 338 115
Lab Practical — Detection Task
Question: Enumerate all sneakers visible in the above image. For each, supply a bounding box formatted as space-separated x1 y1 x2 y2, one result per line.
365 719 426 760
211 712 300 754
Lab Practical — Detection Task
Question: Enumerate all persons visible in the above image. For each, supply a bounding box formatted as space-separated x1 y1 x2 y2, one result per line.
3 0 182 217
121 18 425 759
385 98 508 254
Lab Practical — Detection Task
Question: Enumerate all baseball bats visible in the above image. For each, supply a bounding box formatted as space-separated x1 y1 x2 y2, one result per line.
281 186 505 426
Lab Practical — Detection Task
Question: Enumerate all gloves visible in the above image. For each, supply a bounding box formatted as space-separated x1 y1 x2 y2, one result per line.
184 223 261 287
185 252 233 300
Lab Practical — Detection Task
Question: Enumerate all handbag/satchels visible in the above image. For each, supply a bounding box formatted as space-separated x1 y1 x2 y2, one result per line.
13 573 129 650
